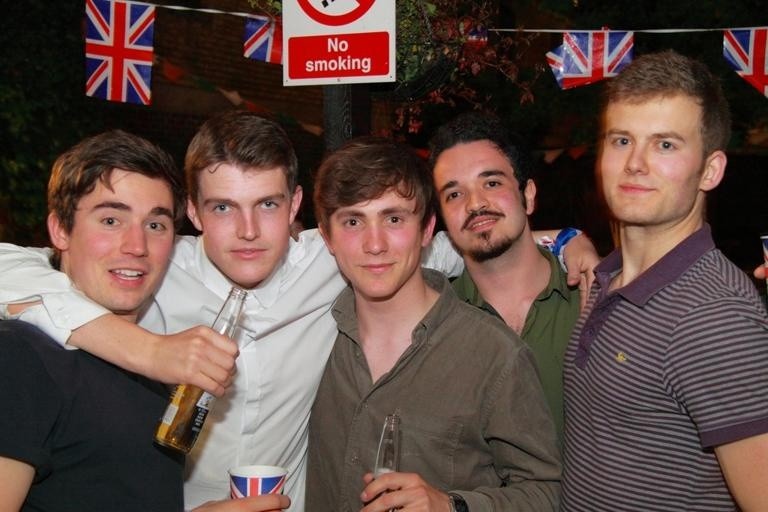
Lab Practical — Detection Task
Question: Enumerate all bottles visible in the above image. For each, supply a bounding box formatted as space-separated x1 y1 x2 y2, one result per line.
151 287 248 455
369 414 401 512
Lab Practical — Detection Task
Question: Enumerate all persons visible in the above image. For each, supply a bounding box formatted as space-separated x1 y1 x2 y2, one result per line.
304 138 562 512
754 236 768 280
428 120 582 468
0 129 292 512
0 109 603 512
561 48 768 512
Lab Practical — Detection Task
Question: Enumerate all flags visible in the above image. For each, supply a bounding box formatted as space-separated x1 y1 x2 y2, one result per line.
84 0 156 106
545 32 635 91
723 30 768 99
243 17 283 66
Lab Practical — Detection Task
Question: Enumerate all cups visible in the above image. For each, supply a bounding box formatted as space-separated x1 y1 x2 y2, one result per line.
228 464 289 500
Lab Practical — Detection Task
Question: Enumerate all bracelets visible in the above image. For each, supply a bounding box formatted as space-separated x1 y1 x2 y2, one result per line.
540 228 582 274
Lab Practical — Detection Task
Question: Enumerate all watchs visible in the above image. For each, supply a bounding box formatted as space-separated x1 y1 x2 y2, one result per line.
448 493 468 512
